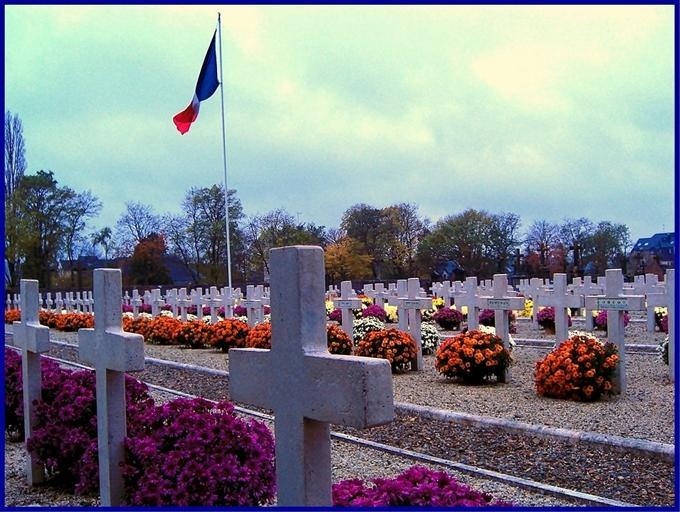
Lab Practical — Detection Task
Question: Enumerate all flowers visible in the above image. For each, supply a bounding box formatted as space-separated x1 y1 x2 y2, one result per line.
1 351 519 512
1 293 673 404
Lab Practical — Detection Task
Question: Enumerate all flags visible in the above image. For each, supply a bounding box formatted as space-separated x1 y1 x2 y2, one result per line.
172 28 220 135
